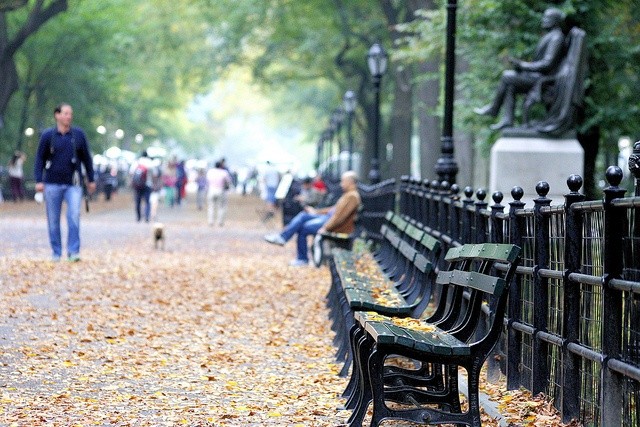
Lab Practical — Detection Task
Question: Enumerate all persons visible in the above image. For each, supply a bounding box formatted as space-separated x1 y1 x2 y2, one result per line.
284 175 321 221
34 105 97 263
129 151 154 222
102 164 113 200
263 162 280 212
154 153 186 207
220 157 233 189
7 151 27 202
261 170 362 264
204 161 233 226
195 168 208 211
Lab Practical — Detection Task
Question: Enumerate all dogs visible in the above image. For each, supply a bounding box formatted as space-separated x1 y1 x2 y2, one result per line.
153 223 166 249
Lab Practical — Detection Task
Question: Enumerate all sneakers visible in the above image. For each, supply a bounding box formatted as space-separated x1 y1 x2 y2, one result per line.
68 255 81 262
264 234 286 246
51 254 61 261
290 259 310 265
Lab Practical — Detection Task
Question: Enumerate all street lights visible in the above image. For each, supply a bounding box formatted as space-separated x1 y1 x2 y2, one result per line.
344 91 357 170
24 127 34 138
317 109 343 179
96 126 106 136
115 128 124 140
365 42 389 185
133 133 143 146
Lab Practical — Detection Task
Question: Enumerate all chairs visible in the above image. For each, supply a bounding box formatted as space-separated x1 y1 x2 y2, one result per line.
335 244 521 427
322 208 444 377
316 205 366 269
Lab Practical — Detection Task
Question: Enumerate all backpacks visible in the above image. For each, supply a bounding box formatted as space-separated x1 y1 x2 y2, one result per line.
132 162 150 190
